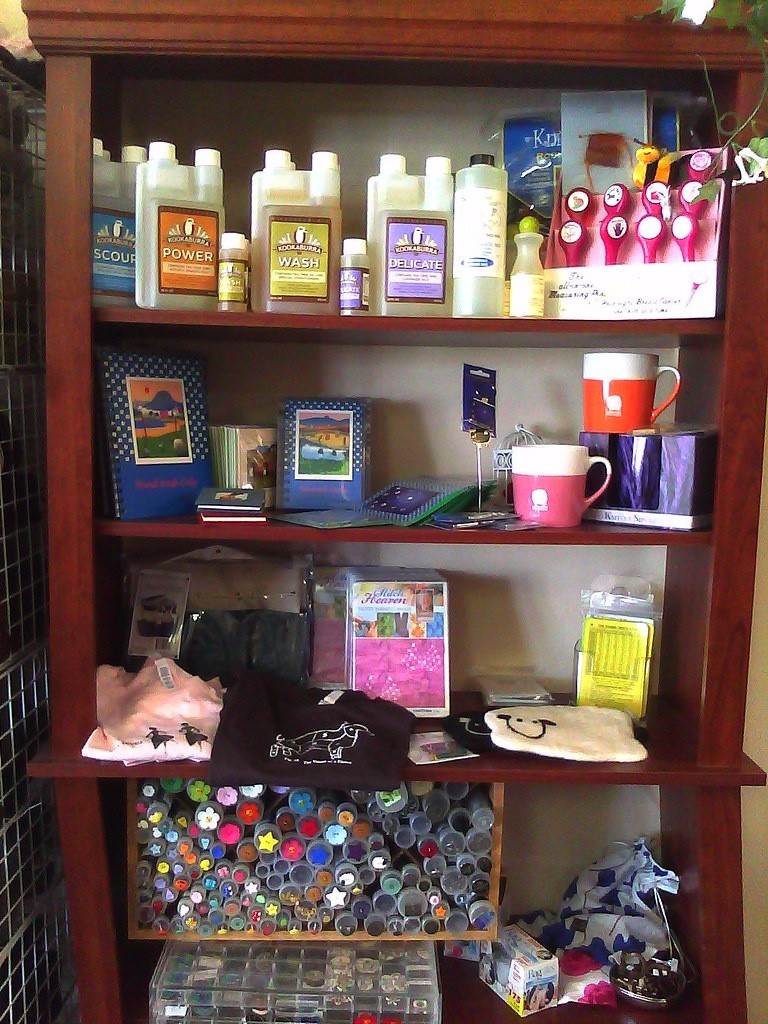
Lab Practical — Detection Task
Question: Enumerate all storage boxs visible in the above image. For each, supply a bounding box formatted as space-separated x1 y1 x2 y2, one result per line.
580 424 715 532
478 922 560 1018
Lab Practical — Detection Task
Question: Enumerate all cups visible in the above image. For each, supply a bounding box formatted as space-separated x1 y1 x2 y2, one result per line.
583 352 681 434
512 443 613 528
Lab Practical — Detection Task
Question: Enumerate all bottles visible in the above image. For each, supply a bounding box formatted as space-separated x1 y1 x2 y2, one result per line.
90 137 545 321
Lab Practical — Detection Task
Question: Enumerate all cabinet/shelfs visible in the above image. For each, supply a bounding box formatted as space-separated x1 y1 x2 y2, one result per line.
23 0 768 1024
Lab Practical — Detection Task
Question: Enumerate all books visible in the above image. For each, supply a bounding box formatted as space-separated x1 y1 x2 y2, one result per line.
314 567 451 718
99 350 371 528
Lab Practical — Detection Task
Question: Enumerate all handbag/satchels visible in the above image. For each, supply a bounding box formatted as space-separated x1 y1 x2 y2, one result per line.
206 665 417 792
80 650 226 766
